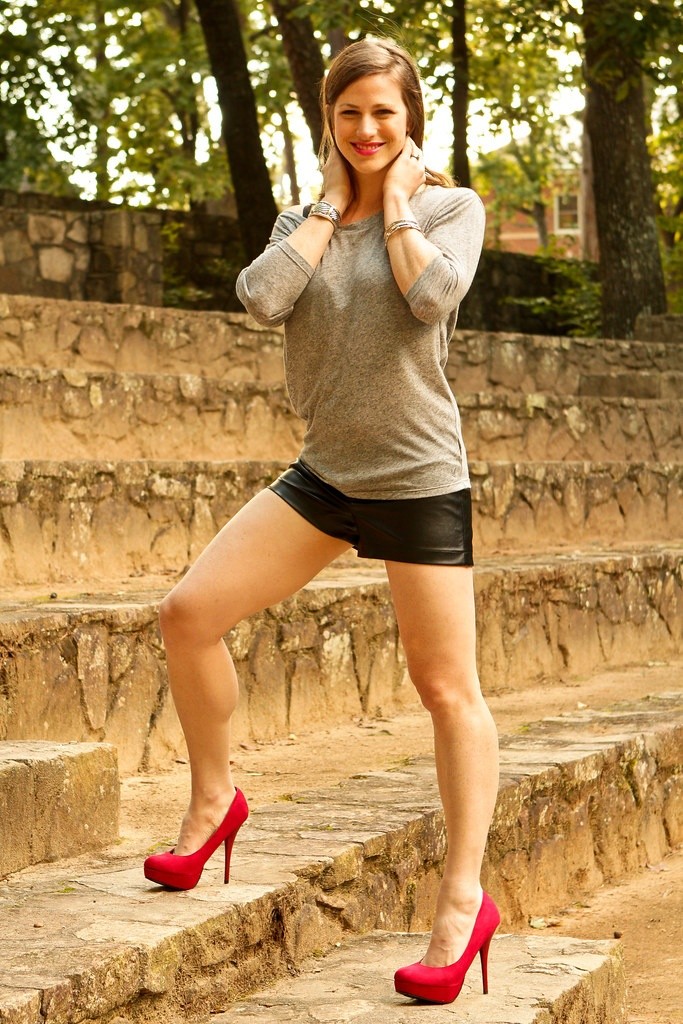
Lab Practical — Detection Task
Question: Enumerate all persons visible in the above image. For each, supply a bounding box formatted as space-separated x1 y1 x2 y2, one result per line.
144 37 498 1004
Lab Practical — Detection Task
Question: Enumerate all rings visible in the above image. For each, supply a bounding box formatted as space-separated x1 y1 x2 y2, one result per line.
412 155 419 160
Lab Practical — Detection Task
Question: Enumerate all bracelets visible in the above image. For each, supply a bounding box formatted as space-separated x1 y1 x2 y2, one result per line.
309 202 341 229
384 220 420 245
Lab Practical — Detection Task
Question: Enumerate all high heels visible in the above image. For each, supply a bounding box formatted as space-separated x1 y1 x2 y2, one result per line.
144 786 248 890
394 891 500 1003
